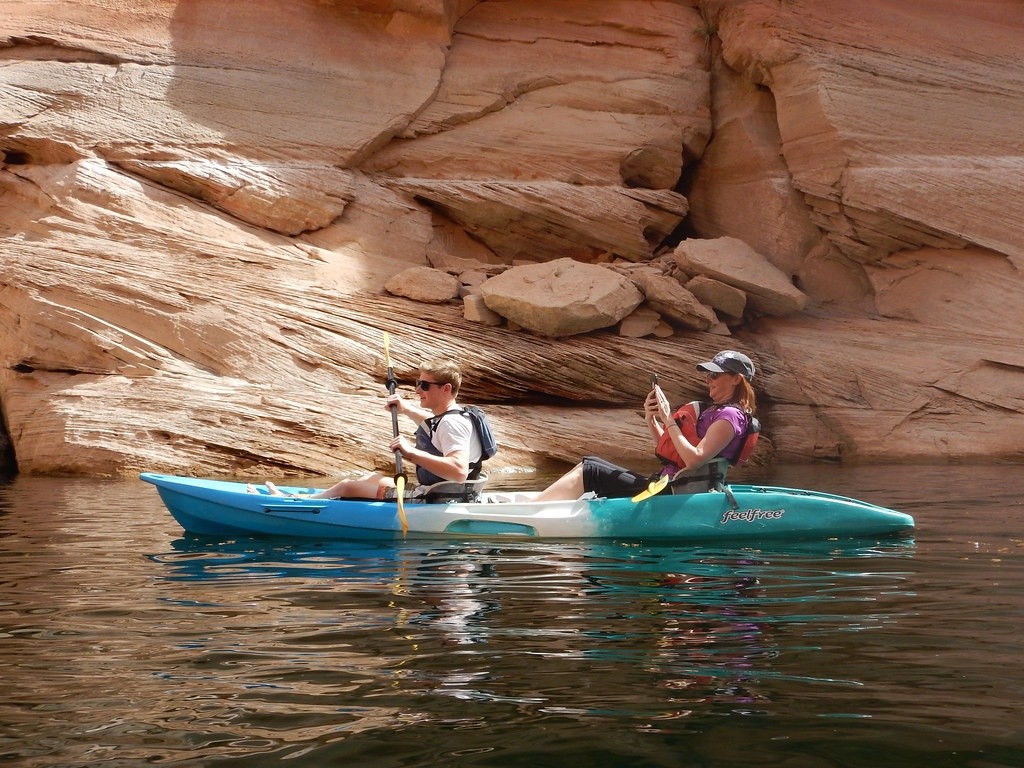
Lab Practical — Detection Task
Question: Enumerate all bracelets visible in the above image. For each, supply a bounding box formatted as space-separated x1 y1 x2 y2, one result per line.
666 422 680 428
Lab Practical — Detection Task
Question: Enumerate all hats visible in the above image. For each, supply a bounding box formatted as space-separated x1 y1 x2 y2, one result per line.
696 350 755 382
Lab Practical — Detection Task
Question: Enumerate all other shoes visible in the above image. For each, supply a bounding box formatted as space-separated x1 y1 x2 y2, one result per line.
487 496 500 503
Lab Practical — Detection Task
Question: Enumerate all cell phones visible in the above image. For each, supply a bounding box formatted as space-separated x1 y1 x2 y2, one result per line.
651 374 658 398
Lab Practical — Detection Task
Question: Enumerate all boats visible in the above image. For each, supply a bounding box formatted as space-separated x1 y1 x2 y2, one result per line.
140 472 917 543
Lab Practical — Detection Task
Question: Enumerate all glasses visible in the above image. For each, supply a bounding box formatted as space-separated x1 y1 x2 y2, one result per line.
416 380 445 391
706 371 726 379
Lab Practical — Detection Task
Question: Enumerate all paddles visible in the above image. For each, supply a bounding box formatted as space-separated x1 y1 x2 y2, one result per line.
631 470 670 503
383 332 409 538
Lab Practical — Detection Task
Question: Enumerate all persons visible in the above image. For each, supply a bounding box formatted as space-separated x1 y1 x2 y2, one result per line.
247 360 486 499
488 351 756 504
